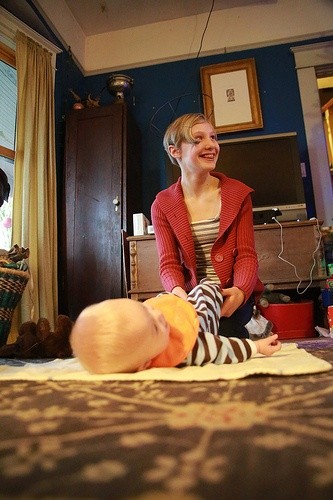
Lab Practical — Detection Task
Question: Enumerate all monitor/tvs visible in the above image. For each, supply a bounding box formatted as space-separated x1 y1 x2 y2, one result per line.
172 131 308 225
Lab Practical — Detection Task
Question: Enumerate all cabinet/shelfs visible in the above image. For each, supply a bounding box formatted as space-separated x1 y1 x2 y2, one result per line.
126 219 331 329
64 101 140 321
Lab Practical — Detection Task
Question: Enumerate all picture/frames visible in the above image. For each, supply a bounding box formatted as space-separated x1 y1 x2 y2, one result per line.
200 58 264 134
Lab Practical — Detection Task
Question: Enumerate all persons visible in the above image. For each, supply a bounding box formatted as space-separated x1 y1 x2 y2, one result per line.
151 113 266 339
69 283 282 374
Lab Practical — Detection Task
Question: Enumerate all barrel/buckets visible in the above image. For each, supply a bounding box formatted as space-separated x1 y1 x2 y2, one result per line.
257 300 315 339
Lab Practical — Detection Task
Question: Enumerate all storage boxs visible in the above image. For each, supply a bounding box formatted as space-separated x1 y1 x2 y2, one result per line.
258 301 315 340
